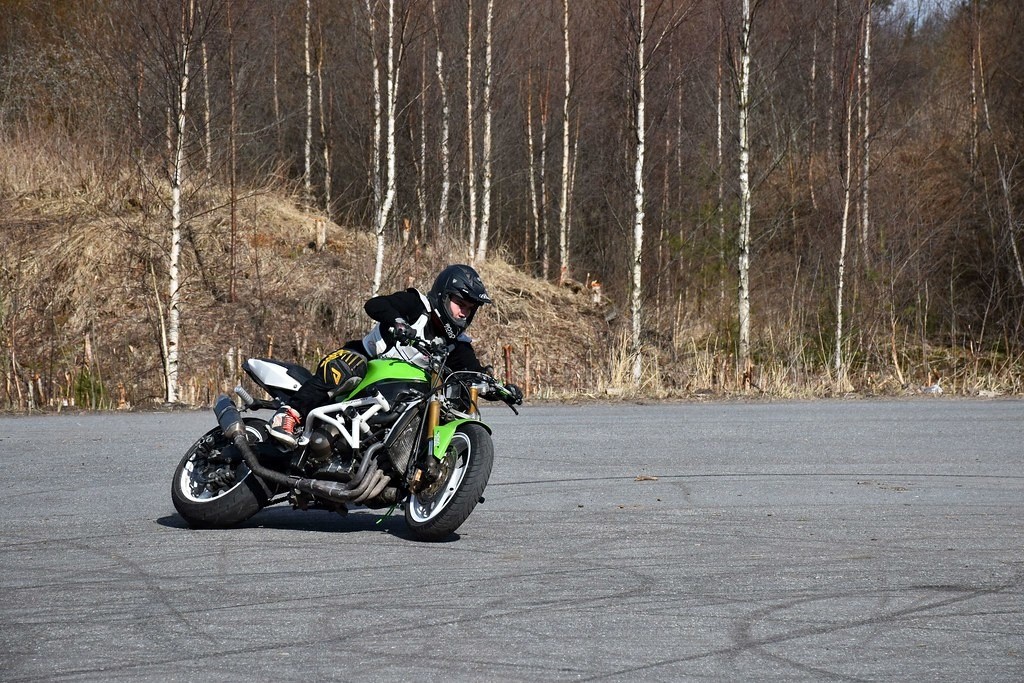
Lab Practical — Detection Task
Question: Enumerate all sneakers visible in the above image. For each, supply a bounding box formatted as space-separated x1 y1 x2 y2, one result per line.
265 406 302 446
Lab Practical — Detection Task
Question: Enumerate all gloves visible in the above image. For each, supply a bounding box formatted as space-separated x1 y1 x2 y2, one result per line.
394 317 417 346
497 384 523 405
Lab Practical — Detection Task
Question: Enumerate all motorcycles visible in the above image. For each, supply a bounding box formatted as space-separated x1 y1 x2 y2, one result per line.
171 327 522 541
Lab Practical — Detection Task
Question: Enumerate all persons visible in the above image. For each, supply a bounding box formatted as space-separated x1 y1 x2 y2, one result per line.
263 264 524 512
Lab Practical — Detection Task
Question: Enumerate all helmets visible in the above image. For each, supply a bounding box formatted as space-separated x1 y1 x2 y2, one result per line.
427 264 492 340
